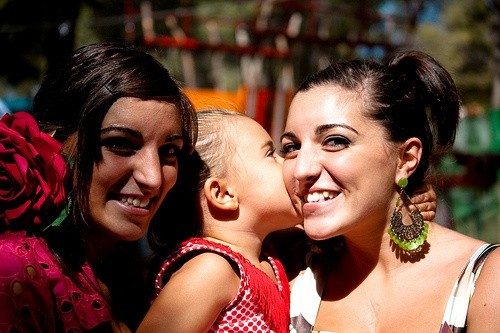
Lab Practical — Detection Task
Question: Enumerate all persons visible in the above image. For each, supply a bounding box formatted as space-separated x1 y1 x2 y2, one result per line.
0 42 500 333
